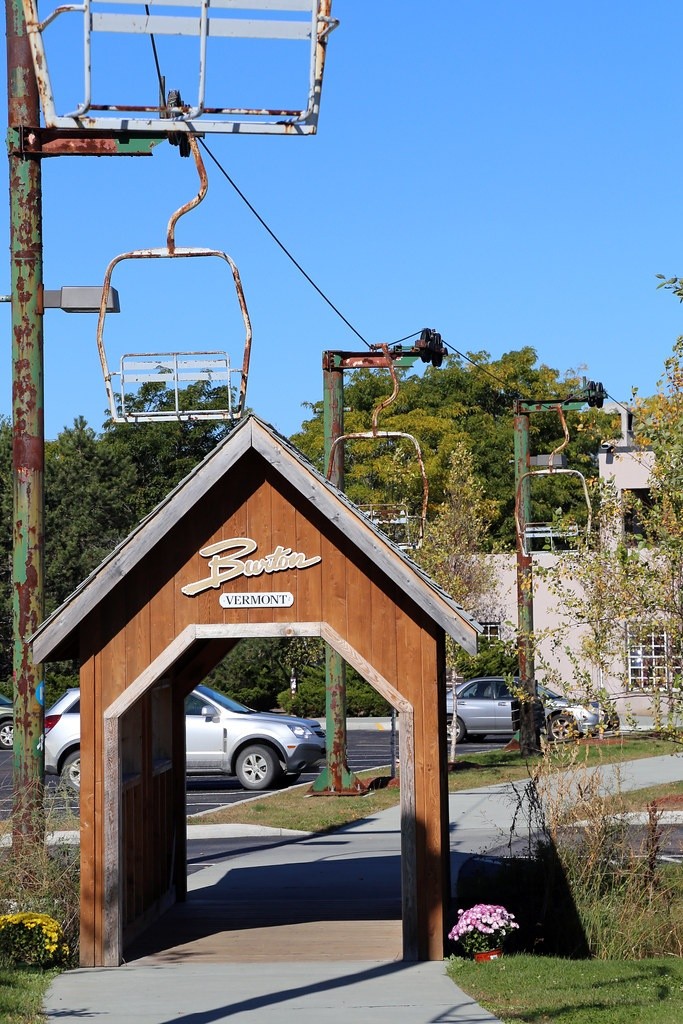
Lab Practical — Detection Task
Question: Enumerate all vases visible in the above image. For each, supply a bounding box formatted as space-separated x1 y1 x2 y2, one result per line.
474 950 502 961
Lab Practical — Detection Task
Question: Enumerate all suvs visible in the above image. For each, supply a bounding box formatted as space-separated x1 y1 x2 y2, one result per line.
44 682 327 791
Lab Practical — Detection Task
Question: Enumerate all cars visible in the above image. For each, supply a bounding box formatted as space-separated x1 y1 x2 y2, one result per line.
446 677 620 744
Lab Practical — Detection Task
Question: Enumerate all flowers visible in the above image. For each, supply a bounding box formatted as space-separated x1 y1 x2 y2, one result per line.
447 904 521 951
